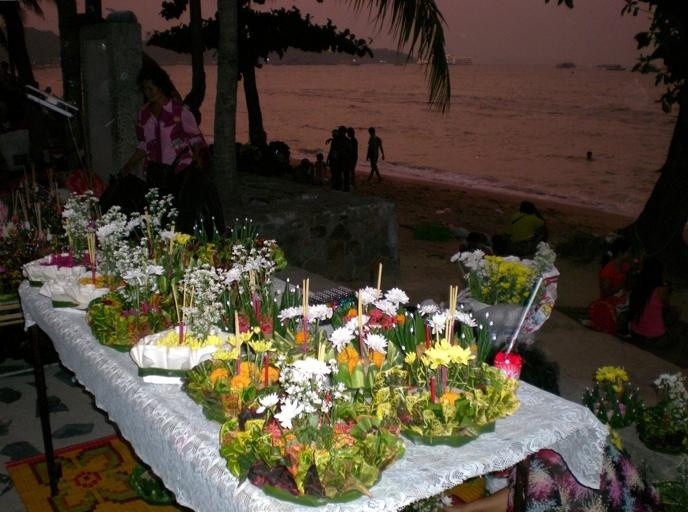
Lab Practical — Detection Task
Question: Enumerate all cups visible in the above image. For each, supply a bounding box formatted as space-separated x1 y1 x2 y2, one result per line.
494 353 524 384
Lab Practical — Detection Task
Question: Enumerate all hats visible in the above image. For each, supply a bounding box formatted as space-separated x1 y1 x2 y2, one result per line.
607 238 632 258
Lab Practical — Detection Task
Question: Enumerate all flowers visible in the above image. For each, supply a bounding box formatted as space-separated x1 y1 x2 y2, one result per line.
643 372 688 455
212 327 277 384
275 304 334 351
581 366 644 429
404 338 478 388
256 355 348 437
450 241 559 311
0 184 62 292
158 216 308 345
96 188 191 327
61 190 99 265
329 285 500 364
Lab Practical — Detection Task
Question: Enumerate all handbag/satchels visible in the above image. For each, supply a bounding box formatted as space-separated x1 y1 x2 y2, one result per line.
185 142 223 188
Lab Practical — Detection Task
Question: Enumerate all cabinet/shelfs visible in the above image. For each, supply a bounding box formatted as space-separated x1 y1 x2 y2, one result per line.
18 275 590 512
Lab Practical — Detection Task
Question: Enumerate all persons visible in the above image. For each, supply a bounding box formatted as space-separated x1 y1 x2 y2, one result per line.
2 143 87 172
599 254 620 297
366 127 385 184
665 279 674 294
347 127 358 185
506 200 548 257
191 110 226 242
611 289 630 313
262 130 312 182
326 130 339 181
586 151 593 159
629 257 687 350
458 228 499 256
118 64 207 196
441 340 662 511
334 126 350 191
2 64 58 141
312 154 325 188
